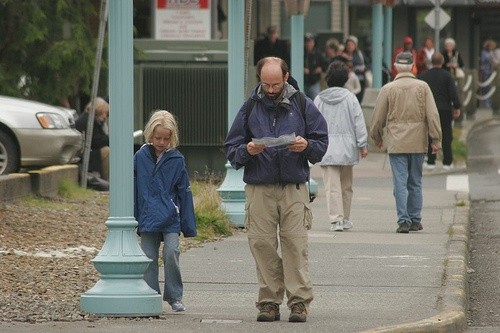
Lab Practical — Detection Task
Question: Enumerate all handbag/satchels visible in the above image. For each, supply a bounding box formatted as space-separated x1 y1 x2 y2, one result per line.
80 172 109 190
364 69 373 88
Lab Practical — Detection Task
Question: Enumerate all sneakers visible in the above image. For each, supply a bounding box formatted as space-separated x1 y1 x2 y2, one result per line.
289 302 307 322
257 302 280 321
168 299 185 311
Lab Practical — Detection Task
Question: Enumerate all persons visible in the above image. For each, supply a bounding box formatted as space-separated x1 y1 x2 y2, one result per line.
370 53 442 234
133 111 197 312
253 25 500 172
313 61 368 232
222 57 329 321
75 96 109 182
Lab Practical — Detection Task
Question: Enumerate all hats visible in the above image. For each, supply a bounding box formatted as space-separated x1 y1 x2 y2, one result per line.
268 26 277 33
396 53 414 64
444 38 455 45
490 49 500 63
347 35 358 47
482 40 496 49
403 37 412 44
305 32 317 40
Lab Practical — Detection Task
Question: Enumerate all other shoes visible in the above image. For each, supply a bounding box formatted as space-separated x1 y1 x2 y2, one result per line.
409 221 423 230
424 161 435 169
444 163 453 170
343 219 353 228
397 221 409 232
329 219 344 231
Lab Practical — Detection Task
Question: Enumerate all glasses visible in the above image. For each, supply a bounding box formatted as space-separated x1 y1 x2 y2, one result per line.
261 82 283 90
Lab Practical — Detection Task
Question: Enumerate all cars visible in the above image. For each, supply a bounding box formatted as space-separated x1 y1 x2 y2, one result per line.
0 95 84 176
